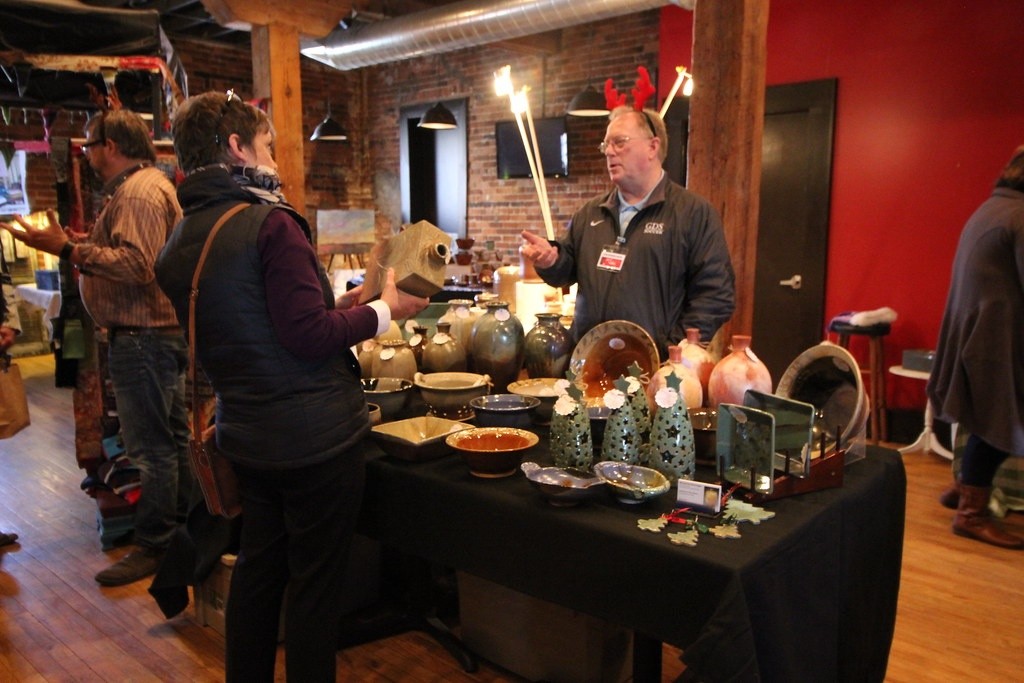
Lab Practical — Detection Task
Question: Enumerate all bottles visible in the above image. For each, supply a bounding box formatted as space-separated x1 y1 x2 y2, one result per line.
597 327 771 485
356 220 574 408
548 374 594 472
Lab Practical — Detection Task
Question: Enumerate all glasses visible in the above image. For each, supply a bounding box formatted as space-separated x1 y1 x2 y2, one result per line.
215 89 242 147
80 137 116 156
598 136 654 153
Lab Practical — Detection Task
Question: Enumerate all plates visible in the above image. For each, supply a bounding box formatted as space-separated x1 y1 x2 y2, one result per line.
717 389 815 495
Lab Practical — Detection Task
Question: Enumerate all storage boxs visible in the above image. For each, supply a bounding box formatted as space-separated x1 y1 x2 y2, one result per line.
35 270 59 290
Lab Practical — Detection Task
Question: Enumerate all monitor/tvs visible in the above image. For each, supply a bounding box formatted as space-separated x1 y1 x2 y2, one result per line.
495 116 569 179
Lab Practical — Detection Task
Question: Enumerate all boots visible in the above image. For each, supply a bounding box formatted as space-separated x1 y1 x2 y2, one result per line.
952 484 1024 548
941 483 961 509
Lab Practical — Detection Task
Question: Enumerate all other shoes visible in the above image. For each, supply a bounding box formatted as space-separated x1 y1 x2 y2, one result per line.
0 531 18 546
95 549 159 585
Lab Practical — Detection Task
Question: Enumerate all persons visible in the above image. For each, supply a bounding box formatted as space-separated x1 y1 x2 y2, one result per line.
157 91 430 683
923 146 1024 549
516 105 737 366
0 109 191 587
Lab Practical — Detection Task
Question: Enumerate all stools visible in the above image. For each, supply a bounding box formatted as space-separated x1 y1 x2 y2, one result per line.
831 315 890 447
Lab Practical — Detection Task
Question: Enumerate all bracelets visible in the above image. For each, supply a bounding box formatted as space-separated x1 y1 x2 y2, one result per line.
60 241 74 260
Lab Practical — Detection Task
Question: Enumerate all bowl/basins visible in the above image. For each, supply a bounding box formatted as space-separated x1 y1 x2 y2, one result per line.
361 320 660 479
776 340 871 447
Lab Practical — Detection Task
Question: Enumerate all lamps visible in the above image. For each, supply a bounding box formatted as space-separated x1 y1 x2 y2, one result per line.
417 53 460 130
491 64 529 115
310 64 348 142
565 21 610 116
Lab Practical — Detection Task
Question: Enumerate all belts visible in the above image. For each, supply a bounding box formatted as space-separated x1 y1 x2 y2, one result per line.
114 326 185 337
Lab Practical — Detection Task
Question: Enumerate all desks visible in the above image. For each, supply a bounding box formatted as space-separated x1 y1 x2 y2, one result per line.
346 279 483 307
16 283 61 353
365 395 905 683
889 366 955 461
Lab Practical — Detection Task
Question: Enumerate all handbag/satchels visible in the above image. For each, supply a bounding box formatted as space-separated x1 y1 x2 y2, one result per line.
0 349 30 440
189 423 242 520
